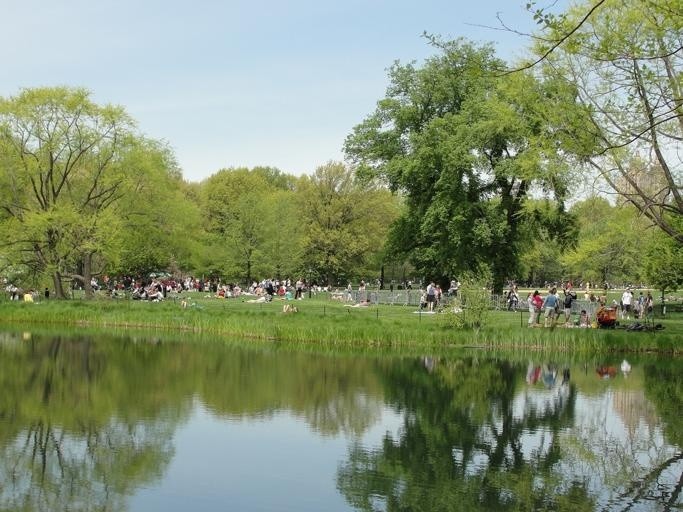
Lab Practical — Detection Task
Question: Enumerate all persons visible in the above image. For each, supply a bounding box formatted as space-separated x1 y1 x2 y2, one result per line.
660 296 666 316
507 279 520 313
90 269 384 313
45 287 49 298
594 358 632 380
390 278 413 291
423 355 438 374
524 358 571 391
3 277 37 303
526 279 655 329
71 281 83 292
420 277 462 314
